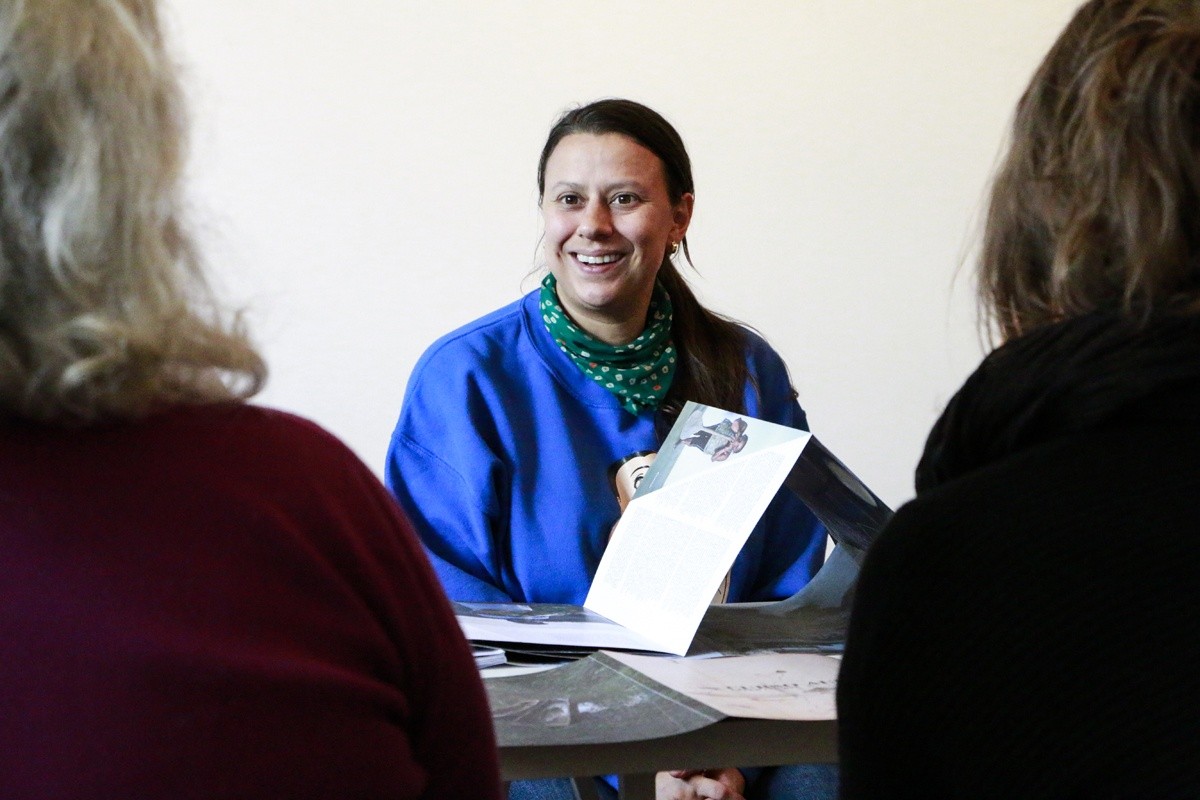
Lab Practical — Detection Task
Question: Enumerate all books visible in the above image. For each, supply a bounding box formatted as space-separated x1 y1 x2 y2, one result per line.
447 400 894 749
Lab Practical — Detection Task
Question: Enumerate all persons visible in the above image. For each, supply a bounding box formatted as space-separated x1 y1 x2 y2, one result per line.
838 2 1197 800
0 0 504 800
386 98 835 798
